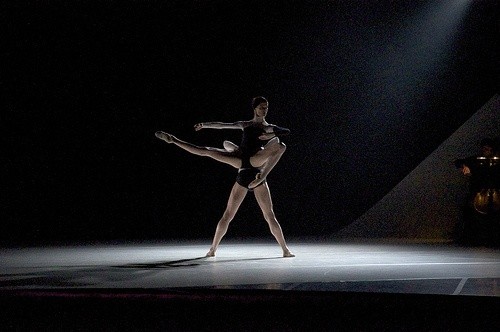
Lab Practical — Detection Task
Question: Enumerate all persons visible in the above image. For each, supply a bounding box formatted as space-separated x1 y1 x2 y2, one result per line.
454 137 499 247
154 96 291 188
204 137 296 258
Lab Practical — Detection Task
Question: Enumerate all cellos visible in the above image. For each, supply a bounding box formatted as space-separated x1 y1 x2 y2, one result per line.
472 150 500 215
